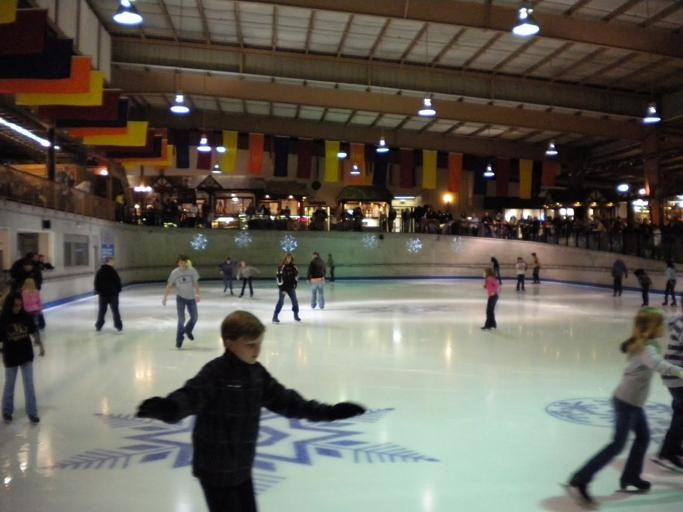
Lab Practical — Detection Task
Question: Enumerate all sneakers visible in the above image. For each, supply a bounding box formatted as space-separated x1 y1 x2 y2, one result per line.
669 303 676 306
566 478 592 502
618 475 650 489
658 447 683 468
174 343 182 347
479 325 496 330
184 330 195 341
271 317 303 322
2 412 41 423
661 302 668 305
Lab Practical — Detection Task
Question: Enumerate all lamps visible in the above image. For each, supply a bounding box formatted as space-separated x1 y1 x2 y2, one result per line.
336 140 347 159
544 59 560 157
374 33 389 154
212 136 223 175
642 1 661 125
350 144 362 176
195 35 212 153
112 1 142 26
510 1 541 37
216 134 226 153
482 100 496 178
415 2 436 117
168 1 191 114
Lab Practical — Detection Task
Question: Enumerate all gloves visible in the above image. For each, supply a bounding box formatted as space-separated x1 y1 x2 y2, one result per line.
136 394 180 423
327 401 365 421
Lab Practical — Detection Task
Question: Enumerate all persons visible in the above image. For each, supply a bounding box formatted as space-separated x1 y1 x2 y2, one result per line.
0 290 47 423
656 293 683 471
135 309 366 511
567 306 682 503
